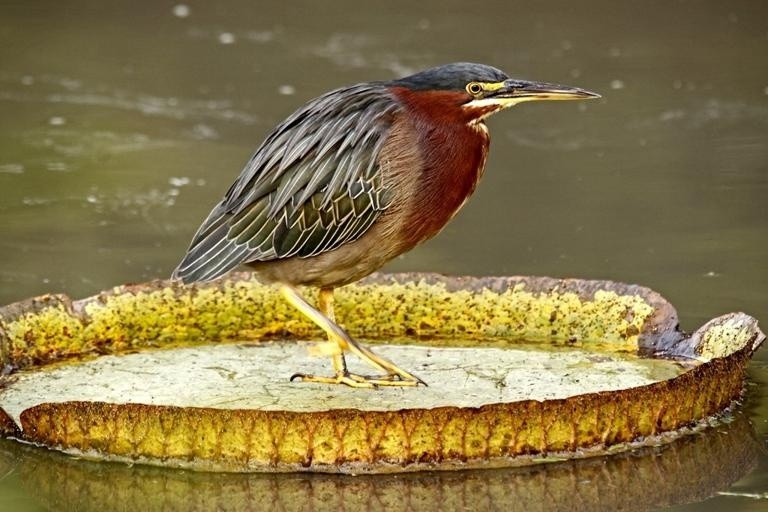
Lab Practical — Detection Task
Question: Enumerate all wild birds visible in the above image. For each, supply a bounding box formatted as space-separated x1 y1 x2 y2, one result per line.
169 61 603 392
